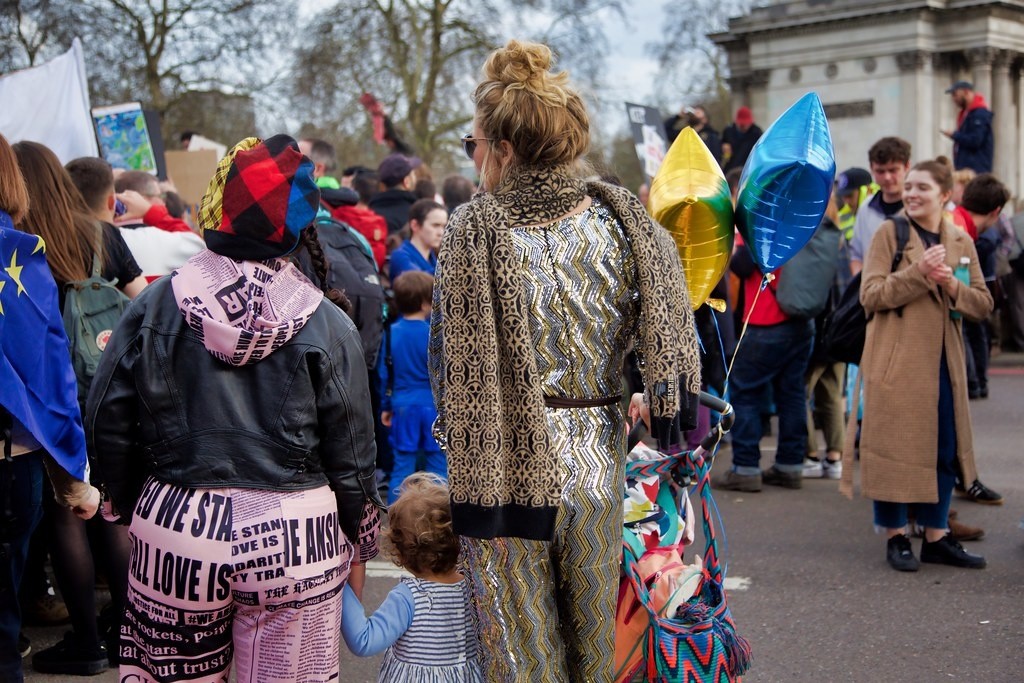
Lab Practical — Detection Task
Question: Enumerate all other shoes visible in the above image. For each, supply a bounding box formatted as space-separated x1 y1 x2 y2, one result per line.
822 460 842 479
801 458 823 477
970 389 989 399
40 602 70 627
17 632 31 658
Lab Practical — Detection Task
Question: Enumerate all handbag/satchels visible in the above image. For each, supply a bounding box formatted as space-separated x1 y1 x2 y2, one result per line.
776 215 841 319
642 586 754 683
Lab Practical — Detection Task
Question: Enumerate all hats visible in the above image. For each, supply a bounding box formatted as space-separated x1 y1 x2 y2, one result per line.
836 167 872 196
377 154 421 186
736 107 752 125
945 82 972 94
196 134 320 260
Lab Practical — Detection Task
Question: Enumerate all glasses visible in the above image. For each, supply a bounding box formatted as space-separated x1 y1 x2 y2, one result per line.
142 194 167 202
461 134 496 160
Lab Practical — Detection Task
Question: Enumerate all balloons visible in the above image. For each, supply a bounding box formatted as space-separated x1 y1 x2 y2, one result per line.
734 91 836 283
649 120 738 318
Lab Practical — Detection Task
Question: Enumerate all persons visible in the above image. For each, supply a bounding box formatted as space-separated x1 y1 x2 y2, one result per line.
625 78 1023 572
83 134 389 683
340 472 482 683
426 41 706 683
0 131 89 683
14 127 480 676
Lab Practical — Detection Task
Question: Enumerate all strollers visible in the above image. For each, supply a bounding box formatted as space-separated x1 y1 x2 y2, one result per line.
621 389 735 579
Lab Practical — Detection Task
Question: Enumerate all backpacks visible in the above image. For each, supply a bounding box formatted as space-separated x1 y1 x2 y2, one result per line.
825 216 910 365
62 219 133 397
294 216 385 370
319 197 387 275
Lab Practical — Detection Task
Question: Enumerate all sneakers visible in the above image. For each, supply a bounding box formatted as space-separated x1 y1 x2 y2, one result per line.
711 470 761 491
32 630 109 675
762 465 801 489
96 607 122 667
887 479 1004 572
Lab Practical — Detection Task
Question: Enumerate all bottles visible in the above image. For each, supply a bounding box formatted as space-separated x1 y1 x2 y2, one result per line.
947 257 970 321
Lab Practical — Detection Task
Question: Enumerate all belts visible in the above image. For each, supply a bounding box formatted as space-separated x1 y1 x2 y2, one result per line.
0 433 42 460
